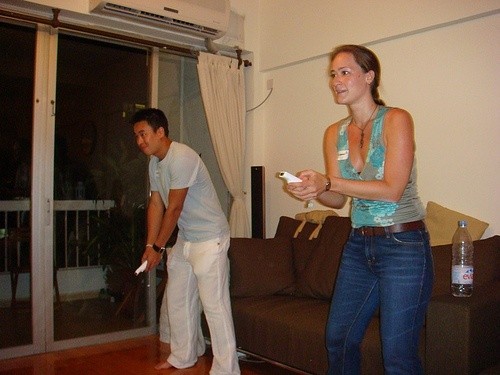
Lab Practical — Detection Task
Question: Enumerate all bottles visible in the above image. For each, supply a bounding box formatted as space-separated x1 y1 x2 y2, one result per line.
450 220 475 298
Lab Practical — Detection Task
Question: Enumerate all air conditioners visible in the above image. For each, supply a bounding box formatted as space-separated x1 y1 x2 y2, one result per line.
88 0 231 41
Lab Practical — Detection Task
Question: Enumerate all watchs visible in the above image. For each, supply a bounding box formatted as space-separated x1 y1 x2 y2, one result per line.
325 175 331 191
152 243 165 255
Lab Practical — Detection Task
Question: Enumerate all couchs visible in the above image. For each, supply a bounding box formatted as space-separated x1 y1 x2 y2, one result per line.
178 216 500 375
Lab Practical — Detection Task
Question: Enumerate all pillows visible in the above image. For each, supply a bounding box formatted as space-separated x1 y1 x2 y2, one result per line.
269 217 324 274
229 237 296 298
422 200 488 247
298 215 351 300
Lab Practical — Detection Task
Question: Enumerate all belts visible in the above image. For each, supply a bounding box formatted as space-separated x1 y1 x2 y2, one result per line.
354 220 426 237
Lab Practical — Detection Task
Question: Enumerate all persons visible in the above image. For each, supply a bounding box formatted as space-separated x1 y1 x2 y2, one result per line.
132 107 241 375
286 44 434 375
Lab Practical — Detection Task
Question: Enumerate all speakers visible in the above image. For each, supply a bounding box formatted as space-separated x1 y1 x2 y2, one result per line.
250 166 266 239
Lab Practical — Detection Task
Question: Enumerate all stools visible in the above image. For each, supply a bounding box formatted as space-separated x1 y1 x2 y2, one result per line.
7 224 61 316
